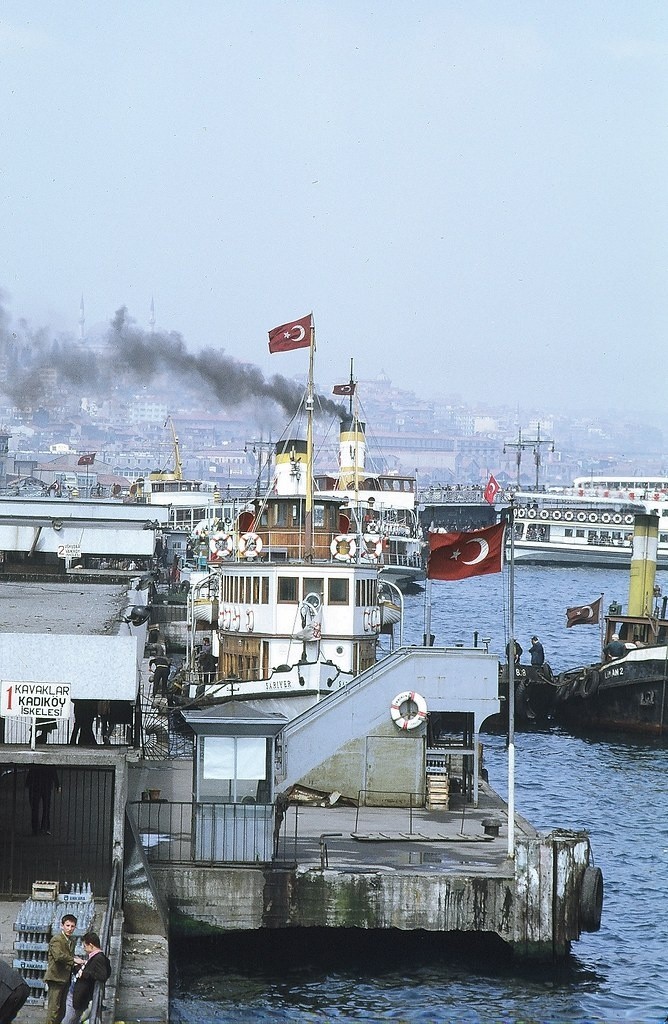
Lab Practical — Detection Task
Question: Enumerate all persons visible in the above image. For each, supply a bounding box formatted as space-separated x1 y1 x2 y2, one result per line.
61 932 110 1024
360 510 381 531
505 639 523 665
407 520 635 567
25 765 61 838
602 633 626 662
44 914 86 1024
148 658 171 696
528 637 544 668
0 959 29 1023
427 481 546 502
198 637 220 685
31 699 119 746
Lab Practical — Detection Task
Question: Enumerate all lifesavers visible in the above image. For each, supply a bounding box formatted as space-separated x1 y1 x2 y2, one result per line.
390 690 428 730
179 579 190 594
580 866 604 933
601 513 610 523
543 663 554 683
589 513 597 522
564 512 574 520
329 535 357 561
239 533 263 557
613 514 622 524
367 523 378 534
518 509 525 517
555 670 600 700
361 535 383 560
552 511 561 519
219 606 254 633
209 534 233 557
514 509 517 516
577 512 586 521
364 606 381 632
528 509 537 518
625 515 634 524
540 510 549 519
515 678 533 716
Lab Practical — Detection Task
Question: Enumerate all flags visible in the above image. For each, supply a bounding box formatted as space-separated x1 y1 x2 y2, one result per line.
484 474 502 505
334 384 354 398
427 521 504 581
265 314 312 356
566 597 601 628
78 452 96 468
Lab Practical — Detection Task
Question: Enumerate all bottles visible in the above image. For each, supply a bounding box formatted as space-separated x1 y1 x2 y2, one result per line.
12 880 95 1008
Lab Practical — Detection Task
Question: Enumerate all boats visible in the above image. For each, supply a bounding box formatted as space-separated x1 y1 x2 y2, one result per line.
500 491 668 571
126 311 425 713
556 514 668 737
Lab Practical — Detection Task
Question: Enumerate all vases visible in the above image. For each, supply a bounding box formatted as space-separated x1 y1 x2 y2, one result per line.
142 789 161 801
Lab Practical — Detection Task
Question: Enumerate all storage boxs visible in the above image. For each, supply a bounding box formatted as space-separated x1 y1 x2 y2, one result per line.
31 880 60 900
425 775 450 812
